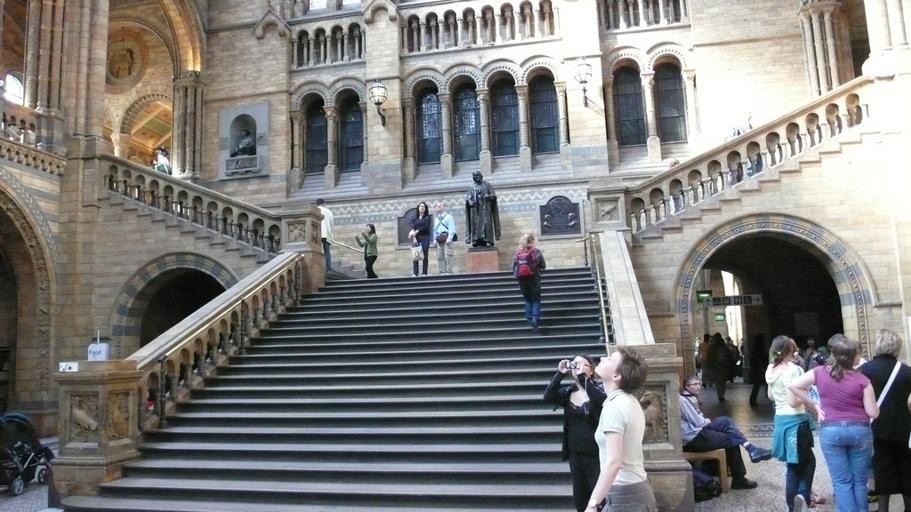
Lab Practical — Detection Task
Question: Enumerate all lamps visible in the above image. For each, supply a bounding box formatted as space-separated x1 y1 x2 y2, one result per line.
571 53 597 110
367 76 388 129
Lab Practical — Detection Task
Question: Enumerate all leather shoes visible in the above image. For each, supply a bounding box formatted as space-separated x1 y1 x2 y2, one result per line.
749 448 773 463
731 477 758 490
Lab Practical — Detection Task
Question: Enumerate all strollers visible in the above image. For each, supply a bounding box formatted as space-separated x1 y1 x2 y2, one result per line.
0 412 55 495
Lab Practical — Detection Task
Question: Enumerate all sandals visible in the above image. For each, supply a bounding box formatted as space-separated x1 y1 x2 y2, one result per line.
812 492 826 504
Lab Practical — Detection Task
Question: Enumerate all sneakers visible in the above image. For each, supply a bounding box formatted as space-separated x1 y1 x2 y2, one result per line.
528 320 539 332
867 490 879 503
411 273 427 277
792 494 807 512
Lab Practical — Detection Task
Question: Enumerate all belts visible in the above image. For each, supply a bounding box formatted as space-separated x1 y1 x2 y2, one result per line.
821 420 870 429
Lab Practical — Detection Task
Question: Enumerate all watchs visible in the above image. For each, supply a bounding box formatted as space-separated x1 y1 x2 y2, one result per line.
588 499 603 510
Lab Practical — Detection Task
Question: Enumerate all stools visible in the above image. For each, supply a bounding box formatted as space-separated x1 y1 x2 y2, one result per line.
682 447 729 493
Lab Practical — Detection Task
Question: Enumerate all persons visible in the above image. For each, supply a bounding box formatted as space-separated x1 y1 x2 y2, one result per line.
512 234 545 332
408 201 430 275
465 170 501 247
233 127 256 156
356 224 379 277
152 146 169 174
433 201 456 272
543 353 604 512
585 345 657 512
315 198 334 273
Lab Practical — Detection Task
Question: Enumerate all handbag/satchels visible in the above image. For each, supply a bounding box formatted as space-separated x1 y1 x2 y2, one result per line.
436 231 458 243
152 163 172 175
410 243 425 261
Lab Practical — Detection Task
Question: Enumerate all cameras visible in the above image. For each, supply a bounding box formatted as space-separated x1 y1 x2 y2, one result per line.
565 361 578 369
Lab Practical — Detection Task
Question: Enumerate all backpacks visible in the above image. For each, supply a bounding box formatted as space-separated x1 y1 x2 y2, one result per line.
693 471 722 503
513 248 540 278
